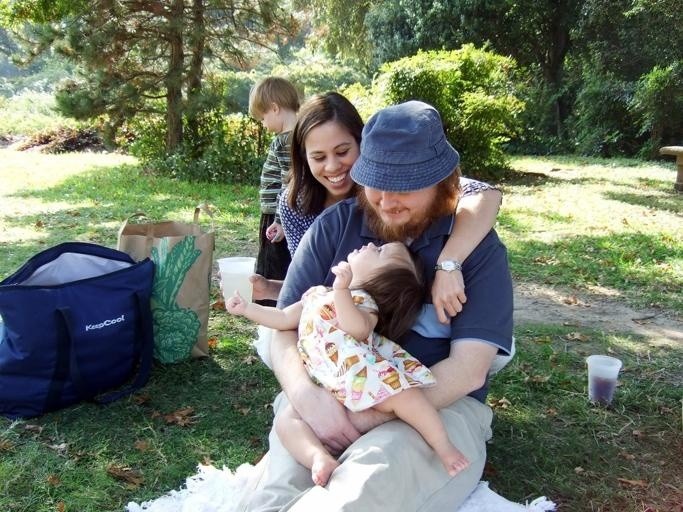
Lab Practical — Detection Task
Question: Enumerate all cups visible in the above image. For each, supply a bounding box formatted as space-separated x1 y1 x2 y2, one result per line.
217 256 256 305
585 354 621 404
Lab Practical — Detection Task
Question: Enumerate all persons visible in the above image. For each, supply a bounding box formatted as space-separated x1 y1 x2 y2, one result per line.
218 238 469 489
212 97 518 512
248 73 304 309
211 90 507 327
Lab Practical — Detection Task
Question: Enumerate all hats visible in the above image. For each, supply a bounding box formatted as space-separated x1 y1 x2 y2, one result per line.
349 100 460 193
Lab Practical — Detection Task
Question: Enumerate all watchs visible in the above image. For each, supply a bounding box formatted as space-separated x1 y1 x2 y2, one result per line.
431 257 463 277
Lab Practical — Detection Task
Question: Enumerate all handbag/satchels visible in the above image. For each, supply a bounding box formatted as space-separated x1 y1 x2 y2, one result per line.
0 242 155 422
117 204 215 365
